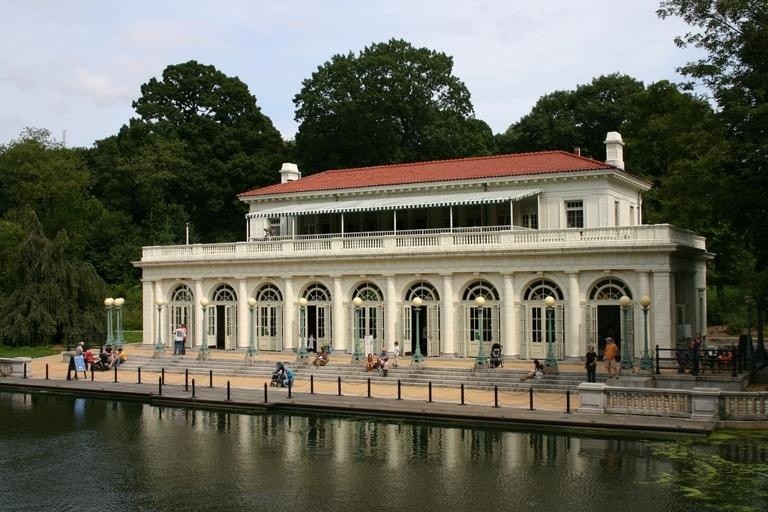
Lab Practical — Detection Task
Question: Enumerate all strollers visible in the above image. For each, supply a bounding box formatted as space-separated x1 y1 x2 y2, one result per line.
488 341 505 368
270 359 285 388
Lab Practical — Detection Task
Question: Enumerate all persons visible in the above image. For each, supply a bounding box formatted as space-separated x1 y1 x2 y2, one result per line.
173 325 187 356
179 324 188 355
693 331 701 374
718 349 736 372
687 336 698 377
391 341 399 366
282 368 294 388
315 350 328 369
383 357 388 376
584 342 600 383
76 340 127 372
307 333 317 354
377 348 389 369
421 322 427 354
520 358 544 382
602 337 620 380
276 369 283 380
366 353 374 373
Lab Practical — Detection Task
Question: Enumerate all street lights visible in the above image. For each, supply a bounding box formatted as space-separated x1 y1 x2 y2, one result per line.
154 297 165 357
472 297 490 369
246 295 259 357
540 295 559 374
616 295 633 375
408 297 425 367
352 297 363 362
637 294 656 371
104 296 127 351
742 292 754 371
297 297 310 360
196 297 210 362
